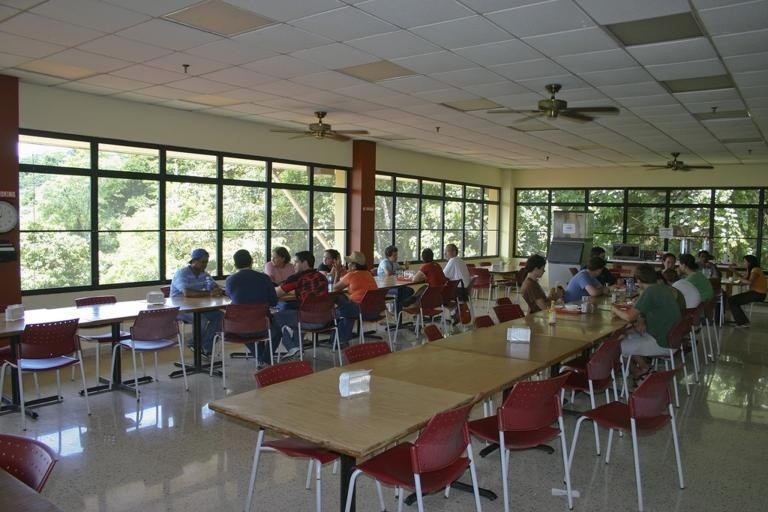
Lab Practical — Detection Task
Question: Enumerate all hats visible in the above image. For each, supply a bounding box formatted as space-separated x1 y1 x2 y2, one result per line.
343 251 365 265
187 249 208 264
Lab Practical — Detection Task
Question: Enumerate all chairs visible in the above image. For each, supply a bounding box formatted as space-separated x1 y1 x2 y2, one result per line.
1 434 58 493
108 306 189 403
244 361 388 512
493 267 721 407
556 337 624 455
344 393 483 512
70 296 146 387
210 302 274 390
465 261 531 312
2 318 92 431
160 286 221 358
340 302 555 446
563 362 687 512
276 279 462 363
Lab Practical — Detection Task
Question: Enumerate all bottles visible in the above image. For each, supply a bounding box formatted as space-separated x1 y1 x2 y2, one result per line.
625 278 634 295
395 269 415 279
612 289 617 302
204 276 212 291
326 273 334 289
548 300 557 325
546 324 556 338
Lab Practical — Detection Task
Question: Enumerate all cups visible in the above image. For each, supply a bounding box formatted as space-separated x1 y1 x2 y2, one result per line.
580 304 592 313
655 254 661 262
604 280 610 287
500 261 504 266
582 296 592 314
729 276 735 282
720 271 726 282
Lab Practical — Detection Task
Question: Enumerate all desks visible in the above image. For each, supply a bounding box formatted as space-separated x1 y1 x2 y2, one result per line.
713 263 751 327
1 294 234 419
207 317 589 507
220 271 427 357
531 282 662 420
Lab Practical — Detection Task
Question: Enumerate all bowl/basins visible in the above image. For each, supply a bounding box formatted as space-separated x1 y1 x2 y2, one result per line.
565 305 578 310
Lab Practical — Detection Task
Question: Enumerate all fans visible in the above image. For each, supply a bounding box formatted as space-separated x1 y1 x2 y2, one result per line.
486 84 619 124
270 112 369 143
640 152 714 172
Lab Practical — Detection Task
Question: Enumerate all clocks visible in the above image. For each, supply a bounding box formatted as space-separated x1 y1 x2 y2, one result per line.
0 200 19 233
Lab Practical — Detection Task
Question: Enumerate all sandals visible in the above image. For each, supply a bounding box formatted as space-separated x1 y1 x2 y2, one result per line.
644 367 657 375
632 372 650 390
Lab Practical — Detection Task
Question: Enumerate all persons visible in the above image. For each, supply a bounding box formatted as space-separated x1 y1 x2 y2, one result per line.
264 246 295 288
318 249 341 276
331 250 379 354
442 243 471 325
224 249 282 372
520 246 767 398
169 248 227 362
376 245 414 316
402 248 446 309
275 251 332 359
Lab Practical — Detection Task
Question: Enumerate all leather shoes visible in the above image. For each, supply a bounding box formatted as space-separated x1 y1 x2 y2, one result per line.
282 346 300 359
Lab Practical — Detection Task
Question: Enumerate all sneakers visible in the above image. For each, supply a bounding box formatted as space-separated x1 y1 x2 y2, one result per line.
255 363 270 373
190 346 208 361
386 302 394 315
736 322 751 328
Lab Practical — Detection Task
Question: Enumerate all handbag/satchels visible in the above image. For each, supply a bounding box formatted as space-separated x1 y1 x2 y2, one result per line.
455 279 470 324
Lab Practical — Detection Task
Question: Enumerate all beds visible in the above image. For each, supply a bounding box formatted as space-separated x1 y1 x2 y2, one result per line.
442 371 573 512
